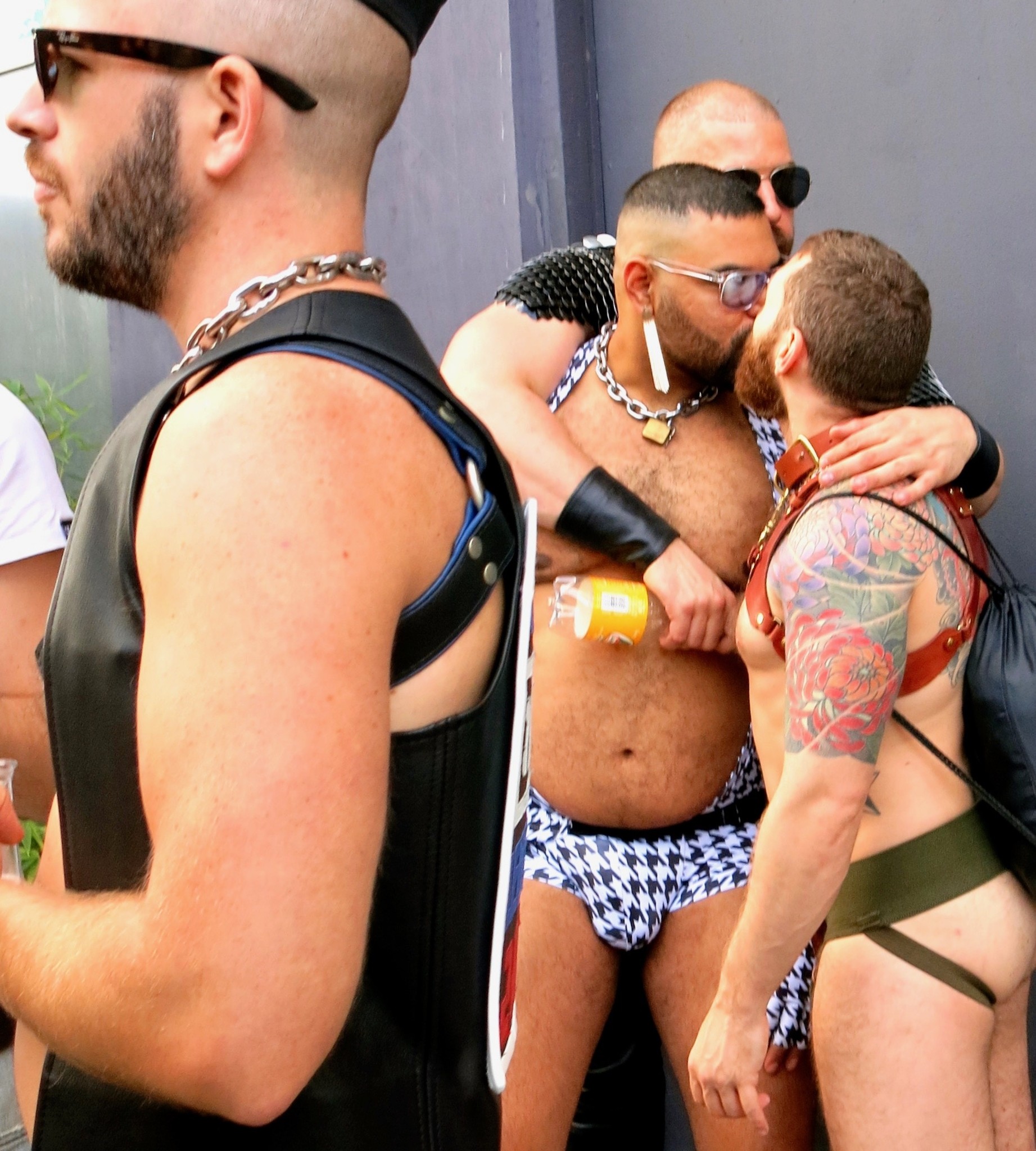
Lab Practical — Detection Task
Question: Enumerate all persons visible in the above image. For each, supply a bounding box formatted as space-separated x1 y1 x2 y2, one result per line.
438 79 1036 1150
0 0 529 1151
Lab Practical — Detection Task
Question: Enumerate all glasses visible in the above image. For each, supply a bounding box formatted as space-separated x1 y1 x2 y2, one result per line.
724 165 812 208
648 259 776 311
33 27 318 112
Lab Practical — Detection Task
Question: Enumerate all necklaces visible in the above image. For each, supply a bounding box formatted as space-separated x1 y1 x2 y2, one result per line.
590 316 733 446
164 250 385 378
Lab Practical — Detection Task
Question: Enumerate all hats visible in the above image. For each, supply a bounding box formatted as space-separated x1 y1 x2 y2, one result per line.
359 0 446 59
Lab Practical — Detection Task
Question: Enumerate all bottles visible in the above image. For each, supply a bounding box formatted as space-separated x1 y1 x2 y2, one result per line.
542 570 726 658
0 759 22 883
